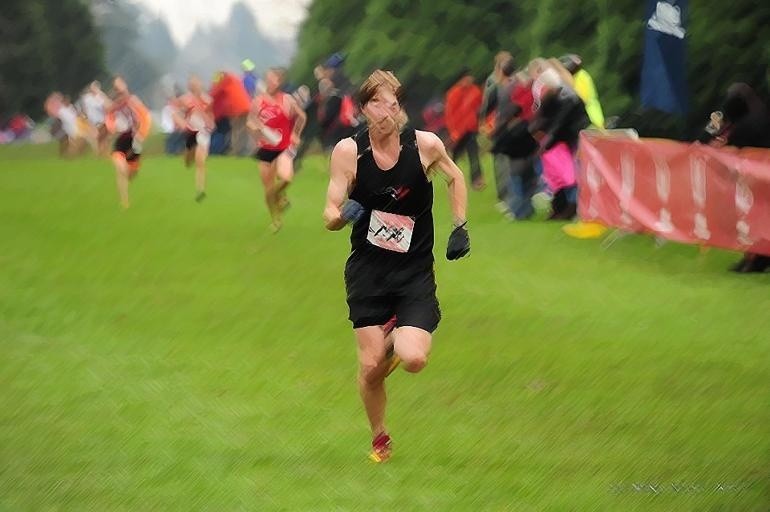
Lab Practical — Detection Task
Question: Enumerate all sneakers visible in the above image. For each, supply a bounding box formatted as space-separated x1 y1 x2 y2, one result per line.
368 431 392 462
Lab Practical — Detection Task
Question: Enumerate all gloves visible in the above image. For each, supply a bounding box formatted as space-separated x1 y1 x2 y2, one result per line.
341 199 364 223
446 221 470 259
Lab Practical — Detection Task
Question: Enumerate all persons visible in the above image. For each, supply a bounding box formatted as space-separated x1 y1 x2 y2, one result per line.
717 80 770 275
10 51 605 230
324 68 473 469
